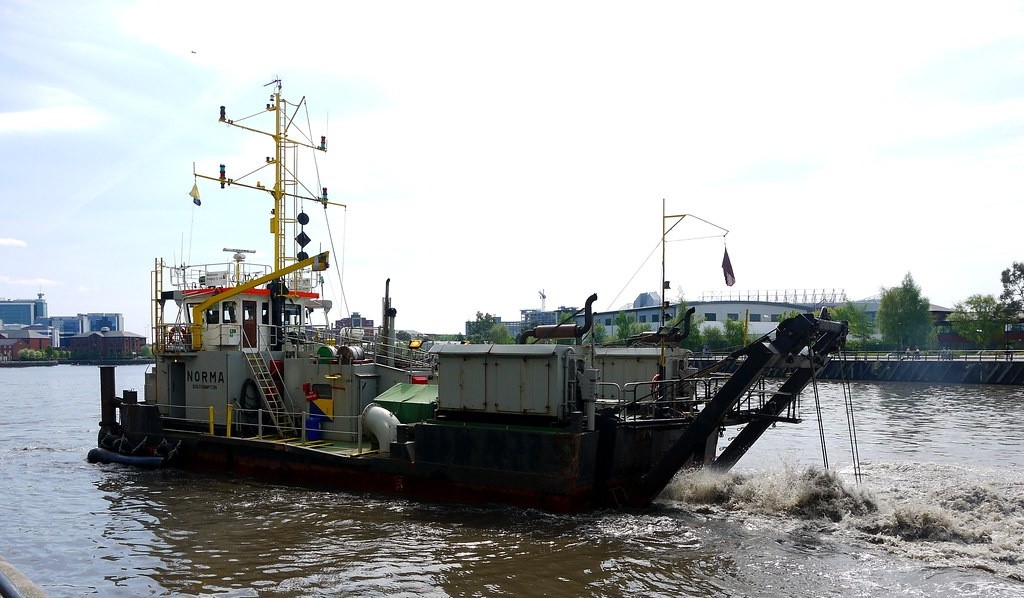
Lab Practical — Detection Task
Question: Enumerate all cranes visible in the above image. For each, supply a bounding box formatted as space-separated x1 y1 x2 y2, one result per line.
538 287 547 311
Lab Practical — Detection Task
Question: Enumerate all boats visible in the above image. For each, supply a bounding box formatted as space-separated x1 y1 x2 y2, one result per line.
139 75 693 516
86 448 165 470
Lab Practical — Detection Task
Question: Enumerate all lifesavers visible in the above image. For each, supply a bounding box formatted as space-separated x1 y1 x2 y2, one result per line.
169 326 187 346
651 374 660 401
